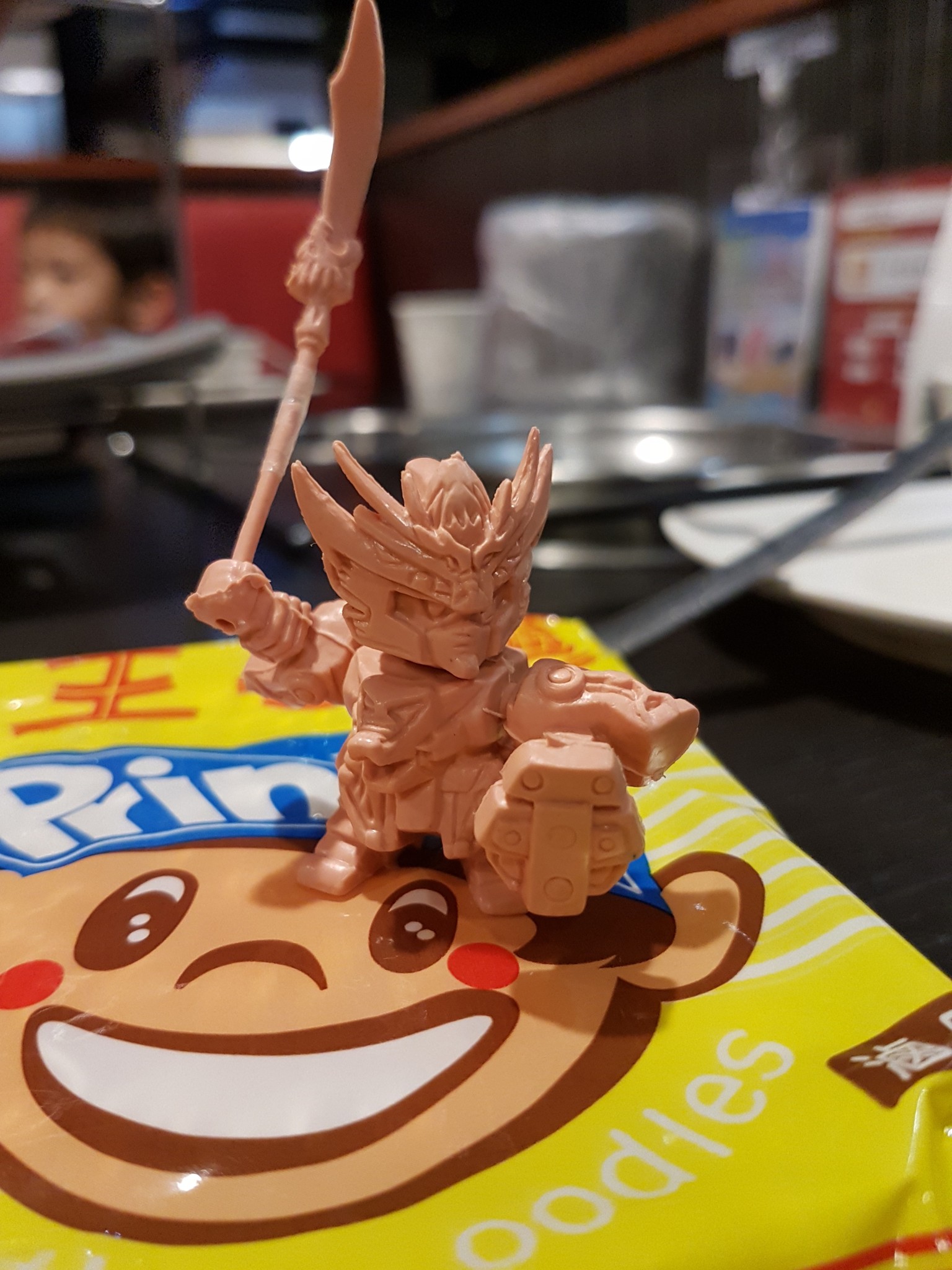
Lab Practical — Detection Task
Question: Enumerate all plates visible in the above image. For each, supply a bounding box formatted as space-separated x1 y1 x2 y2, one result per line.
661 476 951 634
284 402 903 519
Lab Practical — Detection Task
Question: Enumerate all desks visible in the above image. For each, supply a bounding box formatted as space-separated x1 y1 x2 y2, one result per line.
1 409 952 980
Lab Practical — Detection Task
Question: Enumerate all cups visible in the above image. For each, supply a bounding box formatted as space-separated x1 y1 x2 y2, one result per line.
391 297 501 420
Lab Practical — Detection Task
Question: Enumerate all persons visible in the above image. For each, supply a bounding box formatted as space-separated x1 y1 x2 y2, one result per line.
15 177 181 342
